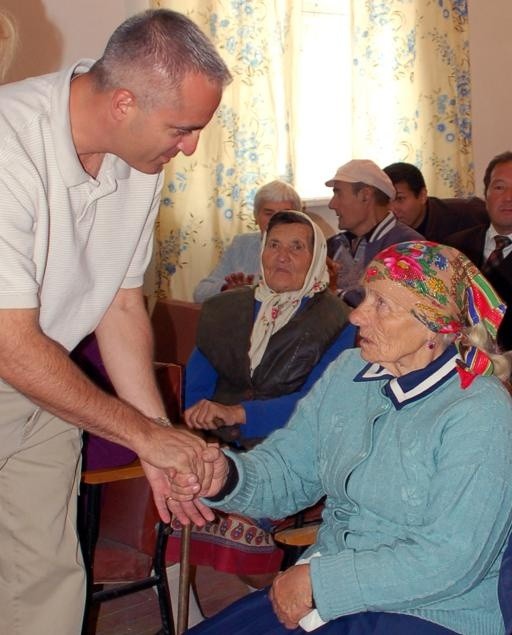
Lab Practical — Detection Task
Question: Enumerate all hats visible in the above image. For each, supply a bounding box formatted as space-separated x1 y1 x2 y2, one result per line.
325 160 396 201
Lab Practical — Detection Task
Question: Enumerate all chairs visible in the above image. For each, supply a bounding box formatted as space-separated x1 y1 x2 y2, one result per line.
80 362 184 633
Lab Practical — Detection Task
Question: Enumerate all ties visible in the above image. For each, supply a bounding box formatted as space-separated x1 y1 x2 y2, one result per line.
484 235 511 274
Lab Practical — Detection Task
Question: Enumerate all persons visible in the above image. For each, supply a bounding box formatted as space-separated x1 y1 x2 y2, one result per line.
382 161 488 244
324 158 429 310
193 180 307 305
438 147 511 318
166 210 364 591
142 236 511 635
2 5 235 635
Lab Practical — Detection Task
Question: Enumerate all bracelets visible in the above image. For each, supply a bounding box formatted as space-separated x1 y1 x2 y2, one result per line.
148 416 175 429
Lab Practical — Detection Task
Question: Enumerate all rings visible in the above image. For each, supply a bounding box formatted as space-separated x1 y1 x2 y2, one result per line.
165 497 174 501
